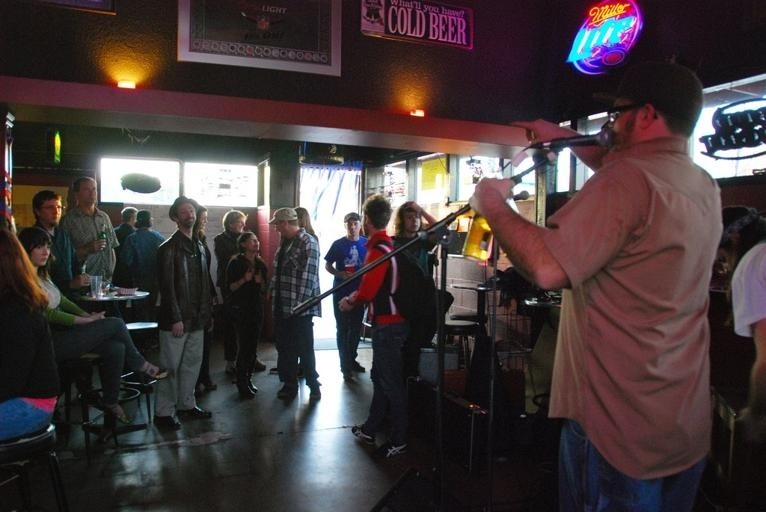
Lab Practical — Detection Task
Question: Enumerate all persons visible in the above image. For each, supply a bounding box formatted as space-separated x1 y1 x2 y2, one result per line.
730 243 764 385
154 197 324 432
467 59 723 511
0 177 166 425
325 191 436 458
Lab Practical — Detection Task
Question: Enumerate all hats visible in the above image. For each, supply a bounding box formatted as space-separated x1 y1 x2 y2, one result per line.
344 213 361 222
169 196 197 221
137 210 152 221
268 208 298 224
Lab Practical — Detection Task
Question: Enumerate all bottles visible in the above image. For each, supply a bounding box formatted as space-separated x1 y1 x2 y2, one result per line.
462 210 493 263
98 223 107 240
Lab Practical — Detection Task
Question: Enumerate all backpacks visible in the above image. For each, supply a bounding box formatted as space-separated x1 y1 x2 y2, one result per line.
376 241 435 320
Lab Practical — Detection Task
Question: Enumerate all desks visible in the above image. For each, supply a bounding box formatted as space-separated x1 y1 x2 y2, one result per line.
72 286 150 447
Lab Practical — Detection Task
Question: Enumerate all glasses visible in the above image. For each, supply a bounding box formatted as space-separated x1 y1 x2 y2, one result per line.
608 103 658 123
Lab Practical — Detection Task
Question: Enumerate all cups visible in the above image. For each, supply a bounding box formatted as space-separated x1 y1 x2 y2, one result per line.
89 275 102 298
102 282 110 297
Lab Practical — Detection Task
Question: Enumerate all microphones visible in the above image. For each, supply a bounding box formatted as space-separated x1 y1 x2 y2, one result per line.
514 191 529 201
529 128 616 148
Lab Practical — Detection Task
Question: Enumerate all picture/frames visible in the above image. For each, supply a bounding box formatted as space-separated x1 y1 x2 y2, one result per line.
34 0 118 15
176 0 343 78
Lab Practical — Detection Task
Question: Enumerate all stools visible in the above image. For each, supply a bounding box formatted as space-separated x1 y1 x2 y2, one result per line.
0 316 161 512
